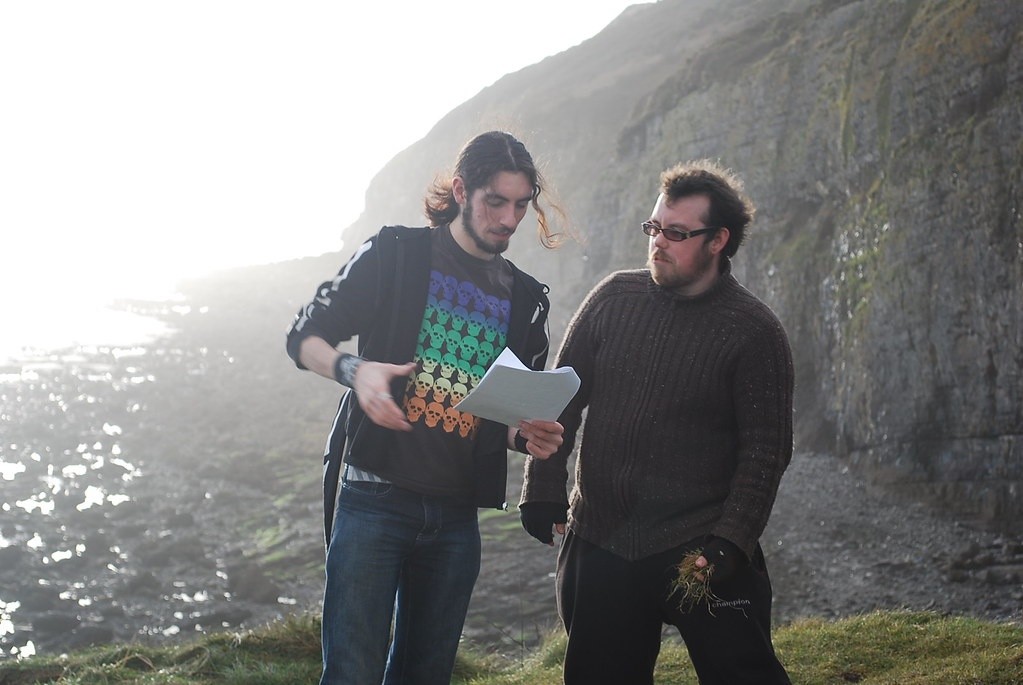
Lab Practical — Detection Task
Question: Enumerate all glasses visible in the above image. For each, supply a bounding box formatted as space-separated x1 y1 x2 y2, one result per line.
641 219 719 242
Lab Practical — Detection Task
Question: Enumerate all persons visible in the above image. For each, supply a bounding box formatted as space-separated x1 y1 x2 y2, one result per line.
518 158 794 684
285 128 566 684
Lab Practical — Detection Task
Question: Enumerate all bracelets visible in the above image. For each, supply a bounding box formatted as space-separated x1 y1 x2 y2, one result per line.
333 351 368 390
512 427 530 455
